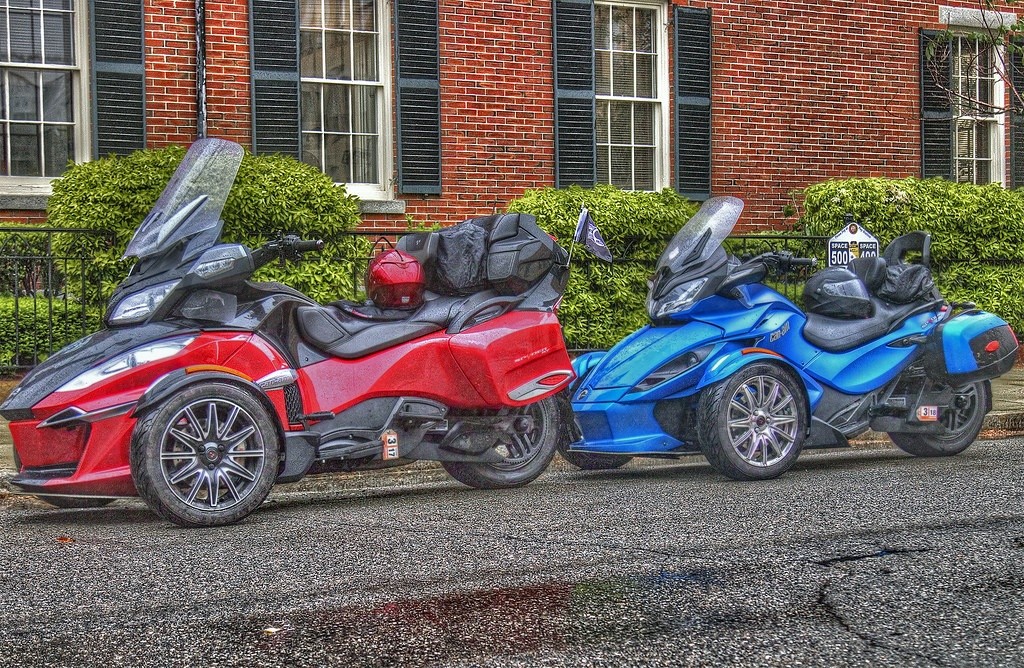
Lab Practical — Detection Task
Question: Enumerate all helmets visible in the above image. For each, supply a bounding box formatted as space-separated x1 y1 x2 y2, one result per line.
802 268 870 320
367 250 425 308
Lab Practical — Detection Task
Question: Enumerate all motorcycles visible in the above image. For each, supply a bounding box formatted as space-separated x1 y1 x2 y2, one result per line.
0 137 571 525
558 196 1019 481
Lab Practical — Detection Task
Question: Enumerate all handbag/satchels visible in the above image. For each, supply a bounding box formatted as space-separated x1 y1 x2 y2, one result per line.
436 223 487 294
878 266 932 302
